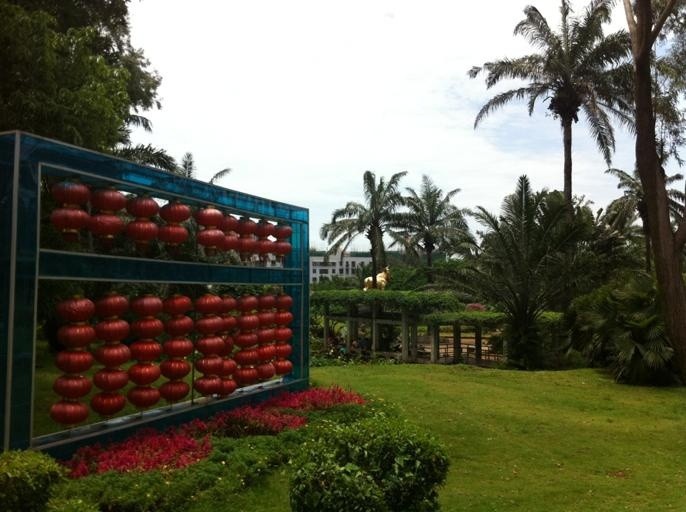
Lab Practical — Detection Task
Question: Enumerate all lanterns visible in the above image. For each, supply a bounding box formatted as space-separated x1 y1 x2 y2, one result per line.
48 181 296 256
47 294 296 425
